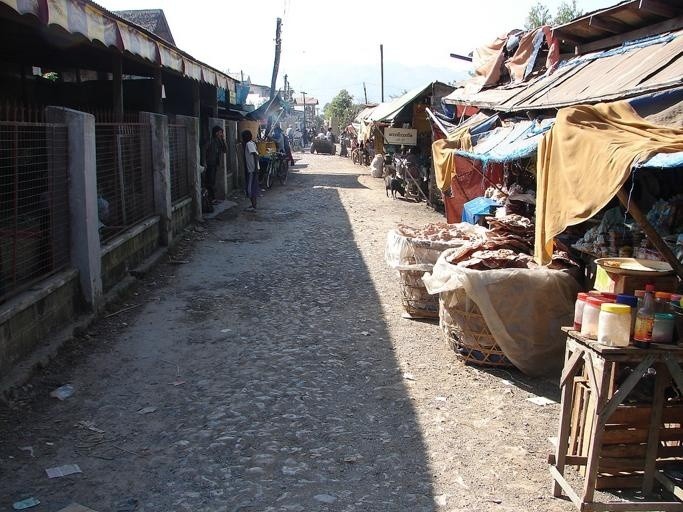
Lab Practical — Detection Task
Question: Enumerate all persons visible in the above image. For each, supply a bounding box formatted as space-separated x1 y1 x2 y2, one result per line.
407 147 418 165
270 126 374 167
201 125 228 204
597 179 645 258
240 129 261 212
512 166 536 218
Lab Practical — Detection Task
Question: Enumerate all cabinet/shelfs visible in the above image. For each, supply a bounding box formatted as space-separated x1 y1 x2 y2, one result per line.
547 327 683 512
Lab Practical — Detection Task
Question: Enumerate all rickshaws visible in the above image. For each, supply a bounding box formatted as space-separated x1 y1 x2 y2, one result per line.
309 138 336 155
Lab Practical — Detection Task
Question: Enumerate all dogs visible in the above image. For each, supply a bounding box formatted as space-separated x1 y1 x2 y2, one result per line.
384 176 405 200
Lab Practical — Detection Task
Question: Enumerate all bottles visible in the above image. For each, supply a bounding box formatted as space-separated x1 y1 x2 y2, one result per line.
572 284 682 348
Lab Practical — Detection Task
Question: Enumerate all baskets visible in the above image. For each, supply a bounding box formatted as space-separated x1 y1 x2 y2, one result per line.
398 224 465 319
438 247 581 368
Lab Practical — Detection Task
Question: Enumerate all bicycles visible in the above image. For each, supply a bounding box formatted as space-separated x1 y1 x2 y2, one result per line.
265 148 290 188
